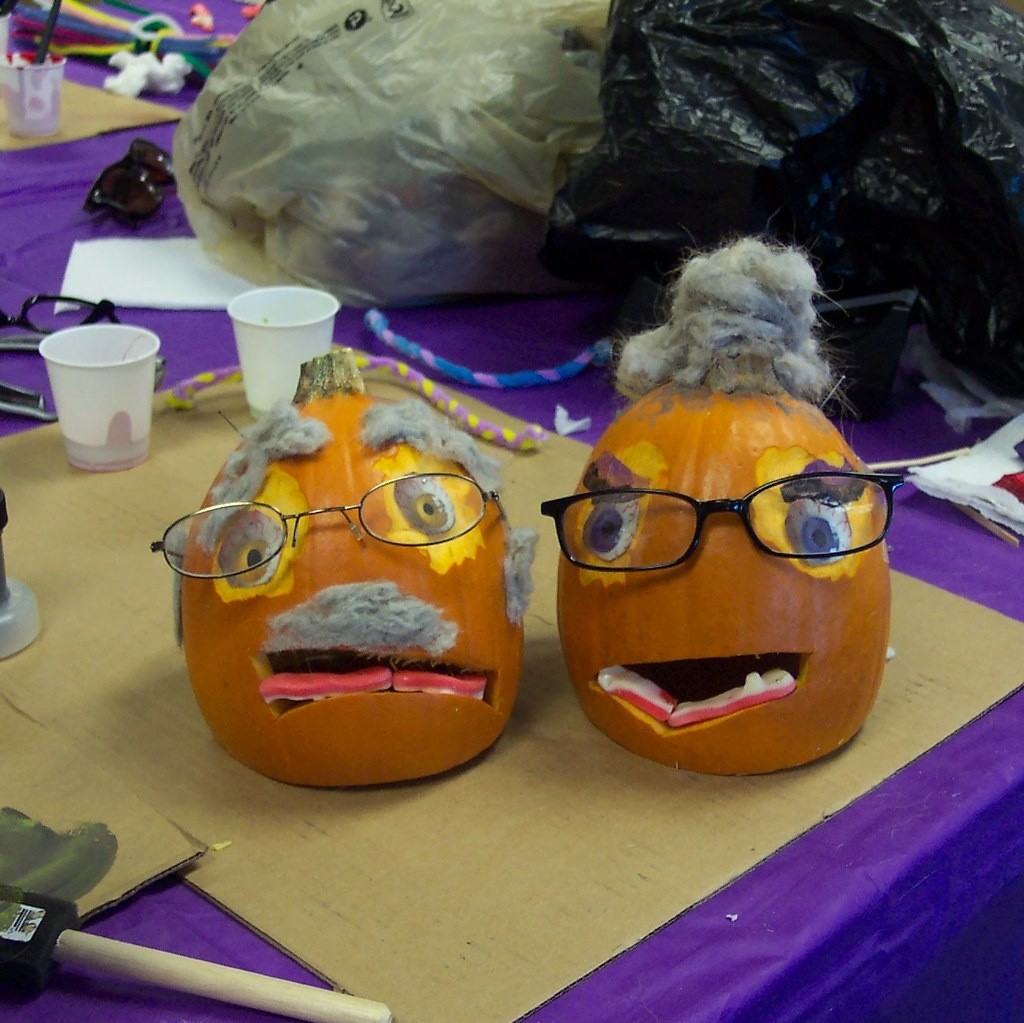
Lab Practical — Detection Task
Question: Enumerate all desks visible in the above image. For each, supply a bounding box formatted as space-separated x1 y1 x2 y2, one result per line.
0 0 1024 1023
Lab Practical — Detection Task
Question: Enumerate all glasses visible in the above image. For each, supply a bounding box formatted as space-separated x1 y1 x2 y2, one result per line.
149 474 497 579
541 472 906 571
0 295 119 350
82 136 176 234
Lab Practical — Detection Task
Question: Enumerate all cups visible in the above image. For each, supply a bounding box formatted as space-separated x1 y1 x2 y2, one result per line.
37 322 161 472
221 285 343 425
2 51 67 140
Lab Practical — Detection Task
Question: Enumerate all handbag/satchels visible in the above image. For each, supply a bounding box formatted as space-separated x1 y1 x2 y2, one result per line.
177 0 618 309
